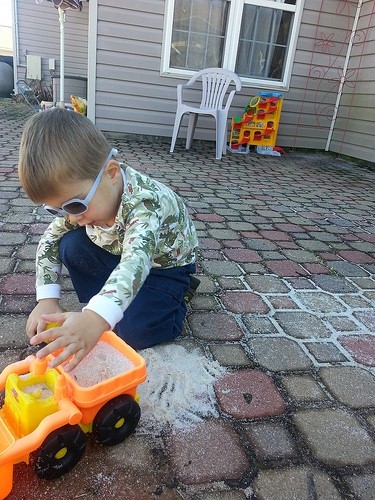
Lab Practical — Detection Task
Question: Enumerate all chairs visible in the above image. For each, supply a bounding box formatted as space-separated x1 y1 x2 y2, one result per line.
169 67 242 160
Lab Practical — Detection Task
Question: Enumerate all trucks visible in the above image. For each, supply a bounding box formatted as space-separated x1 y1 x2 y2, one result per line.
0 325 148 499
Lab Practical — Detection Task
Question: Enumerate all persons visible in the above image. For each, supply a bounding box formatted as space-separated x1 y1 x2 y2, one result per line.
18 107 201 373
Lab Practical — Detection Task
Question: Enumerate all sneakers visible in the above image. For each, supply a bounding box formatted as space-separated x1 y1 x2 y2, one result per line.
184 275 201 303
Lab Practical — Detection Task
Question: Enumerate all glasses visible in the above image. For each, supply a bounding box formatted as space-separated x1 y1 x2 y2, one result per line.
42 147 118 217
